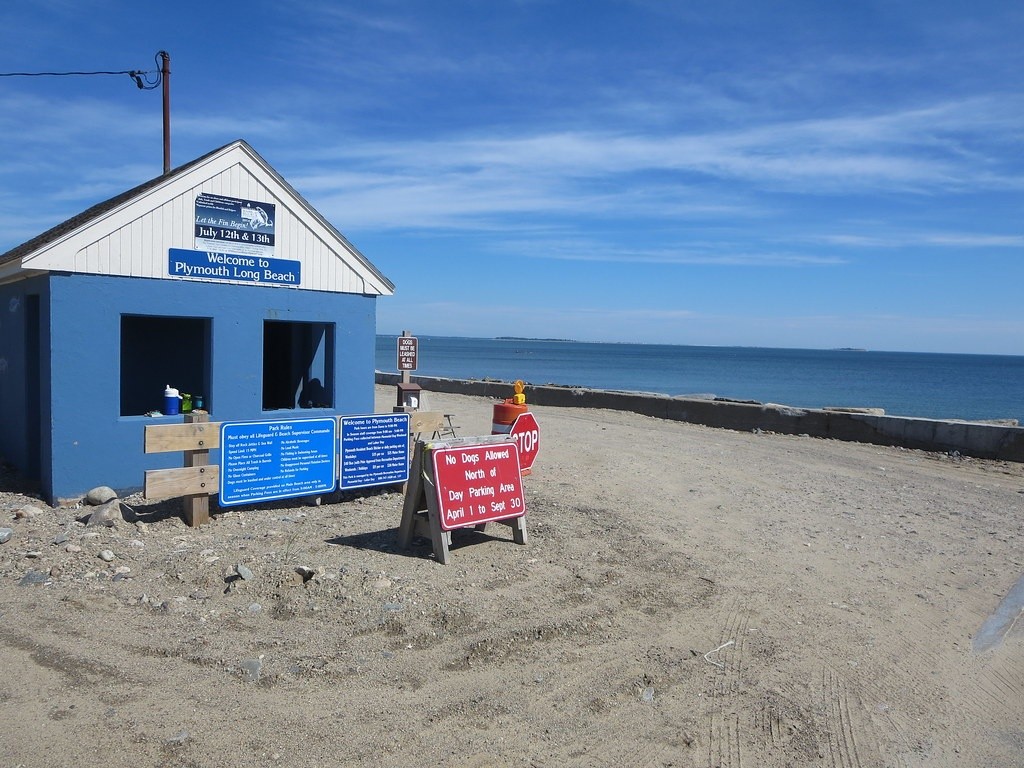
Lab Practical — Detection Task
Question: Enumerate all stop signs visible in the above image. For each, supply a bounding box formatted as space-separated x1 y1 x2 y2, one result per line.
510 412 541 472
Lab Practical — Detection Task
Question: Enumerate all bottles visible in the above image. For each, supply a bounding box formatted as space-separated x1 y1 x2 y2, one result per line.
164 387 182 415
181 394 192 414
